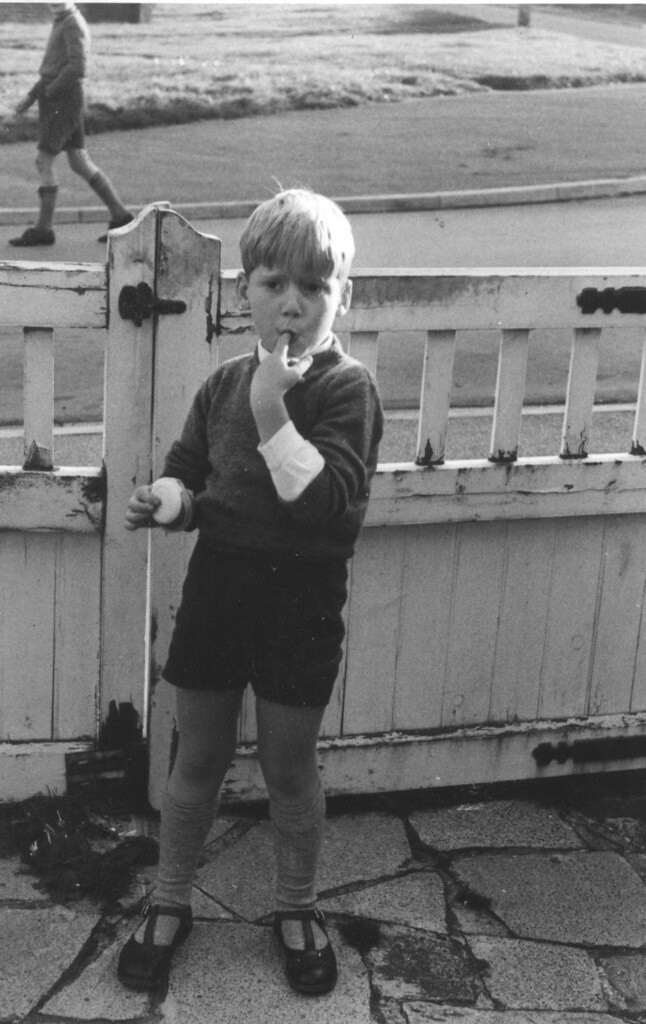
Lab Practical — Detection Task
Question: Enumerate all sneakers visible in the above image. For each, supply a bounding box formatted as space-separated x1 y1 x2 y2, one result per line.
97 214 134 242
7 227 57 247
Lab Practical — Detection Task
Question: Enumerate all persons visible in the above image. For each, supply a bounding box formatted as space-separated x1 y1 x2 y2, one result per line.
8 4 133 247
118 186 386 998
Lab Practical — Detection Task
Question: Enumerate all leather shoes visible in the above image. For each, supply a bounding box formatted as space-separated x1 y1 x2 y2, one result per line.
119 904 193 988
275 909 338 993
